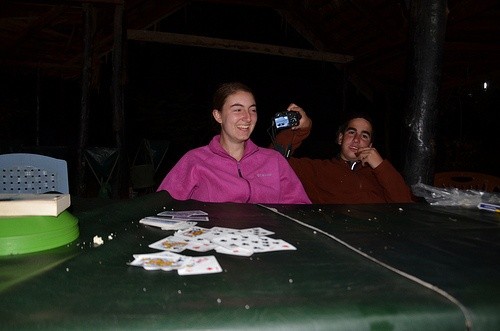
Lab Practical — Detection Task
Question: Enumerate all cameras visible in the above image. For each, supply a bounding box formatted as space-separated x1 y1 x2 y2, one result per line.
274 111 299 130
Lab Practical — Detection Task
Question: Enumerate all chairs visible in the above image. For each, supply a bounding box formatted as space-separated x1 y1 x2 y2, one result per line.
0 152 70 195
435 172 500 193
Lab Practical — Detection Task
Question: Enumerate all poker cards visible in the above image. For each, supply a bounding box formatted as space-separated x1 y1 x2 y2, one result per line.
125 208 297 276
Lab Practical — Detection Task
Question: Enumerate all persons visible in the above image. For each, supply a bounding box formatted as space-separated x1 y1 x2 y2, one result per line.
269 104 416 204
156 83 313 204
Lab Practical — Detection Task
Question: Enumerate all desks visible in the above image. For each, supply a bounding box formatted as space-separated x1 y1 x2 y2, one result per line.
0 190 500 330
